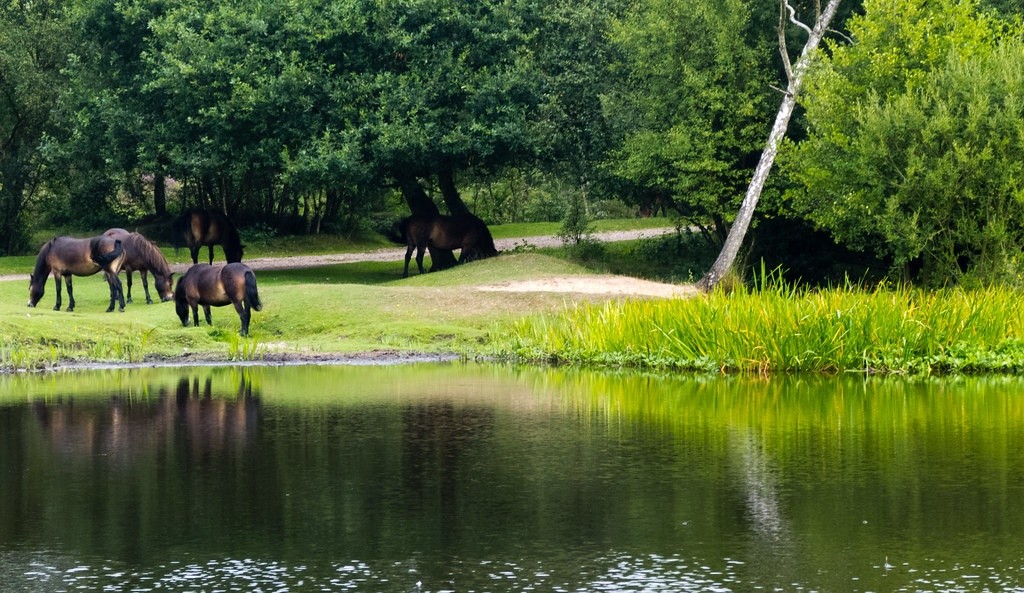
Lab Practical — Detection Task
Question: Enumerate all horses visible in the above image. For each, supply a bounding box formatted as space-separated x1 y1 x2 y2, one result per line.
380 210 502 275
25 210 263 338
